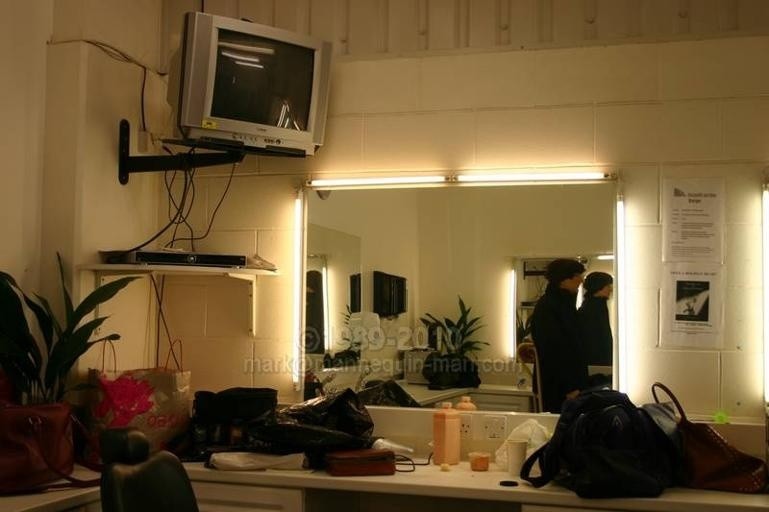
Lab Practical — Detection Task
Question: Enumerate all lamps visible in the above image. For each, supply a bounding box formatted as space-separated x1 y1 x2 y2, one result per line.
306 176 452 187
594 251 615 262
456 170 613 183
290 188 301 393
510 268 515 360
617 183 627 393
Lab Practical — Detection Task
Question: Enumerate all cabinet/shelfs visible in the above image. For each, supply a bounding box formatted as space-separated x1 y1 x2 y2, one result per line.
73 481 304 512
419 389 537 414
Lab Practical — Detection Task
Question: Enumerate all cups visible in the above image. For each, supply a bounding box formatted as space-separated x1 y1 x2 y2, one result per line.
468 452 491 471
506 439 528 474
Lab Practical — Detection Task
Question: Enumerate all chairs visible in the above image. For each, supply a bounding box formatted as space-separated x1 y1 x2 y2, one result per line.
97 427 199 511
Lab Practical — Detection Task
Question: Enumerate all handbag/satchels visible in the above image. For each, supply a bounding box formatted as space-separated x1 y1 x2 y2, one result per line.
0 402 107 492
651 382 765 494
327 449 395 476
86 337 192 466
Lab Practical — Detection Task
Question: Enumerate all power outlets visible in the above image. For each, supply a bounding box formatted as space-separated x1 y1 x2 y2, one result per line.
460 414 473 440
483 415 507 442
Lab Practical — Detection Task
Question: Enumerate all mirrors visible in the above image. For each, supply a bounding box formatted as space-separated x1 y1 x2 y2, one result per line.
306 222 364 372
304 188 616 413
515 255 612 367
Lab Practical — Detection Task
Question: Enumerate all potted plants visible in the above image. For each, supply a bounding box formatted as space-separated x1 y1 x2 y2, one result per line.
418 294 491 390
0 252 143 491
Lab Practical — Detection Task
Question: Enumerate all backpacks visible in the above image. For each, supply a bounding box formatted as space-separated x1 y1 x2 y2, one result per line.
521 388 673 499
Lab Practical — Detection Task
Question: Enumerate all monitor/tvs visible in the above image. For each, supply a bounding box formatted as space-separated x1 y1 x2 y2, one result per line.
372 266 411 316
178 10 336 155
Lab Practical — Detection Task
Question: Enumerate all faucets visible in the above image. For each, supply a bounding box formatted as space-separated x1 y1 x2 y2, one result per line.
355 367 373 393
316 372 336 395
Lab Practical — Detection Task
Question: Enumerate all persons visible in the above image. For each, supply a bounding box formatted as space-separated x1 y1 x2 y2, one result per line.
529 258 585 414
572 272 615 392
